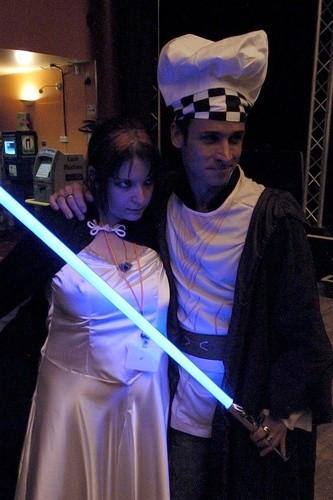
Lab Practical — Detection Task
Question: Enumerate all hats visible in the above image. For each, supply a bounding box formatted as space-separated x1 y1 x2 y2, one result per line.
156 29 269 124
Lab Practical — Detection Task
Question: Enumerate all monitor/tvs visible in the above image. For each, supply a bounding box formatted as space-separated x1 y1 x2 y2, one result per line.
3 140 16 155
35 163 52 178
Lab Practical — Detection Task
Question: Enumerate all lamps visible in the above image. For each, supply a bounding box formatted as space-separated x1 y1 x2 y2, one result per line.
39 83 60 93
20 100 37 107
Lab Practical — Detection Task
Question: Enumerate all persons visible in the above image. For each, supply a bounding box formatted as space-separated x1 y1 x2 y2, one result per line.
0 118 171 500
48 30 333 500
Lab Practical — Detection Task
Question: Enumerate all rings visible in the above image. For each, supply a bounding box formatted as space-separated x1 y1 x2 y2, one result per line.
265 434 275 444
262 424 271 434
66 194 74 199
57 194 66 198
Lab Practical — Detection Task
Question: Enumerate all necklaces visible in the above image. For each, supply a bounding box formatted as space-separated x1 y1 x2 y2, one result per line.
106 240 133 272
